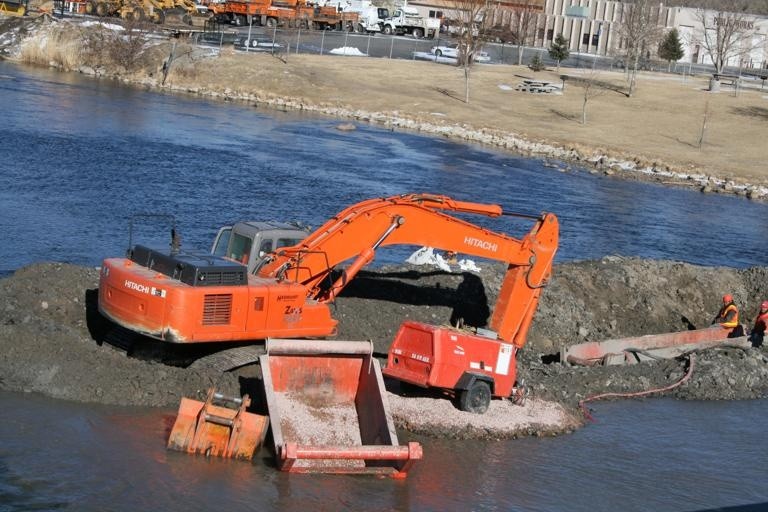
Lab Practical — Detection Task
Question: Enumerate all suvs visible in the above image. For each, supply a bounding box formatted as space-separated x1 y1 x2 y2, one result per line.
441 21 479 41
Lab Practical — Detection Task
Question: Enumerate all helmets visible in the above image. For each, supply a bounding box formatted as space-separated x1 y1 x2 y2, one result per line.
723 295 732 302
760 301 768 308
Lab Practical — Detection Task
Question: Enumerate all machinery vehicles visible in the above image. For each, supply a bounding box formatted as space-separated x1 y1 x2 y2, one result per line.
84 0 197 26
206 0 436 40
0 0 27 17
95 188 560 378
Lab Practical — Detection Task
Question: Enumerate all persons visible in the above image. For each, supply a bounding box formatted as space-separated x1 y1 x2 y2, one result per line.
753 301 768 335
713 294 740 329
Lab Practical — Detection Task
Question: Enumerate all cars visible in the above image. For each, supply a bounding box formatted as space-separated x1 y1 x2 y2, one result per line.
478 24 520 45
430 42 491 64
612 56 660 72
